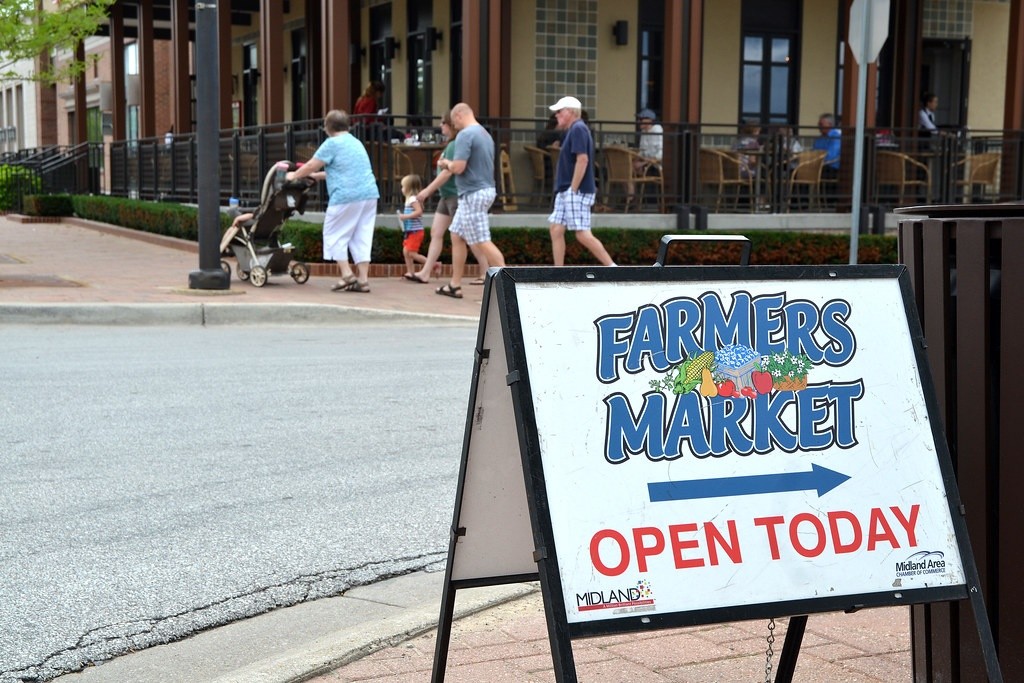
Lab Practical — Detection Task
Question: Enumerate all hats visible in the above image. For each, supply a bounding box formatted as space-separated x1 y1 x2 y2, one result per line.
549 96 582 111
636 108 655 119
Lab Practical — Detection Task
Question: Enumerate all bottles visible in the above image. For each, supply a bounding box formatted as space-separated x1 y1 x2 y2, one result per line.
404 134 412 145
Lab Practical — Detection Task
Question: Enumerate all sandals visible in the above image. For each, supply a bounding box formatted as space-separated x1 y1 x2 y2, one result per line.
331 275 357 291
435 283 463 298
345 280 371 293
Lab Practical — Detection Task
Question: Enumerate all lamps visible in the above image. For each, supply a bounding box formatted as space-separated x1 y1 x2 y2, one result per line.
425 27 442 50
248 68 261 87
296 55 306 76
613 20 628 45
384 37 399 59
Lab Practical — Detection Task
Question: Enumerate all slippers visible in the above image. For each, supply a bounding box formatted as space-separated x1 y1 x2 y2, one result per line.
469 278 485 286
403 272 429 284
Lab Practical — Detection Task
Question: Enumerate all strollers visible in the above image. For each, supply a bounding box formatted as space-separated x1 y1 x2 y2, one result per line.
220 158 317 287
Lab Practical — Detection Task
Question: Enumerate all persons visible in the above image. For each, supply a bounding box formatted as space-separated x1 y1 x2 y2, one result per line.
352 80 391 130
811 113 841 179
536 96 620 267
398 173 442 280
230 108 380 292
915 93 956 203
626 108 663 194
402 111 491 285
435 103 505 298
774 125 802 179
404 118 423 140
732 121 767 213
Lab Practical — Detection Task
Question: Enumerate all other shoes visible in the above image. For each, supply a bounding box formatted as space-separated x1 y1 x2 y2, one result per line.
433 261 442 278
759 203 771 207
593 204 615 212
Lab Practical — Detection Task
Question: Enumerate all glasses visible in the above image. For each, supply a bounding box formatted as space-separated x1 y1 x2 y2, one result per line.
640 117 649 121
557 109 570 114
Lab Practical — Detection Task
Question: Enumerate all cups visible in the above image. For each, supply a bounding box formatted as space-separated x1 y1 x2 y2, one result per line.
435 134 441 144
229 197 238 210
410 129 419 141
442 136 449 142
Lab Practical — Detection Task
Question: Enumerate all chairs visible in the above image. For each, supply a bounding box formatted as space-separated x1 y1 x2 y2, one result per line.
229 152 260 200
699 146 839 215
364 140 414 212
952 152 1002 204
876 149 931 205
296 148 315 162
523 144 666 214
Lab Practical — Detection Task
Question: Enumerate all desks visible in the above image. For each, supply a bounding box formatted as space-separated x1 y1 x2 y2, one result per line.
395 141 446 176
900 150 965 203
739 148 800 211
